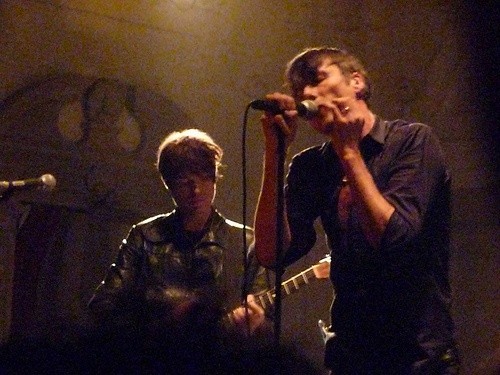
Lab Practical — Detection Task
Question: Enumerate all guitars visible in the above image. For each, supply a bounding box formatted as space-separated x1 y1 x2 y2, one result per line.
223 251 331 325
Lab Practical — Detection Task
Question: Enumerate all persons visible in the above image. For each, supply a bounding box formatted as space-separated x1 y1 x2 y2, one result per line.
252 46 461 375
88 127 264 338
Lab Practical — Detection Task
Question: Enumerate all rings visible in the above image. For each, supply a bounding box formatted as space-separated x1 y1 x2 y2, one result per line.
343 105 351 114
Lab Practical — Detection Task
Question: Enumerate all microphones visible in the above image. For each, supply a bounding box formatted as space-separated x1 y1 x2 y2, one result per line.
0 173 57 192
250 98 319 121
